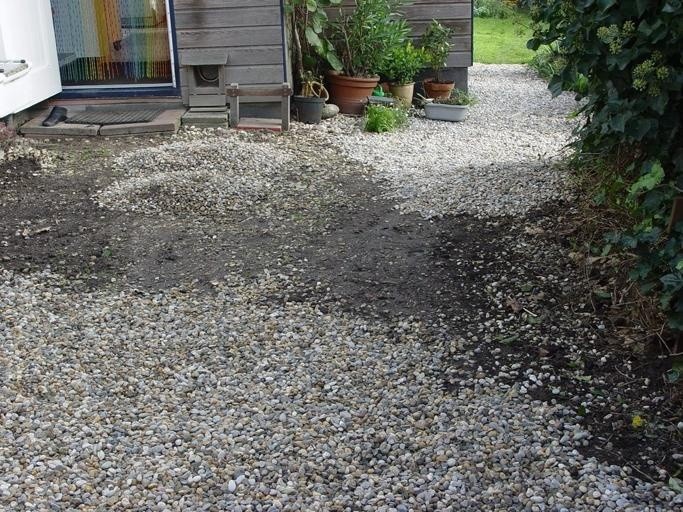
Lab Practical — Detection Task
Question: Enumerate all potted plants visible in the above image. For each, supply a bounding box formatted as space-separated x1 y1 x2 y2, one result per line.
293 70 325 124
422 88 470 121
417 17 456 99
385 39 434 106
324 0 414 114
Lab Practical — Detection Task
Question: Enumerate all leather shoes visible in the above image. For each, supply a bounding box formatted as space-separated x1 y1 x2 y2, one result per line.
43 107 67 126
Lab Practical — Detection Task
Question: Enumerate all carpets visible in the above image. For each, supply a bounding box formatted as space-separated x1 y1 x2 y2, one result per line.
65 108 166 125
